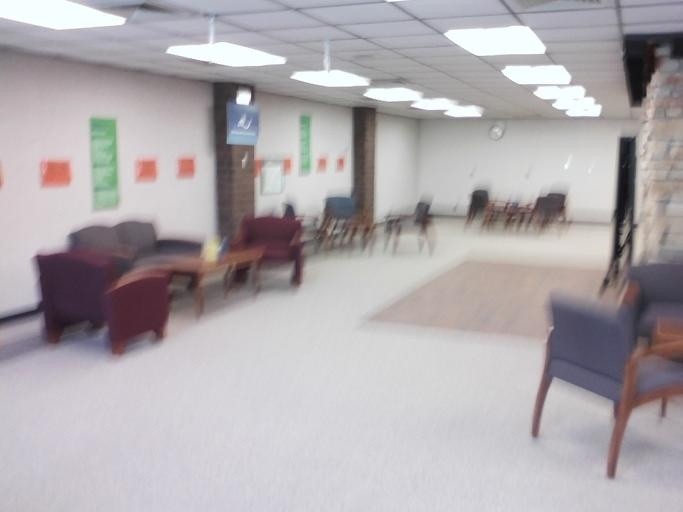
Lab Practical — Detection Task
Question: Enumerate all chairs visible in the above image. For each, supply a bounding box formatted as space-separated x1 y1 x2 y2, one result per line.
529 260 683 479
464 188 568 230
31 213 307 357
287 196 433 250
